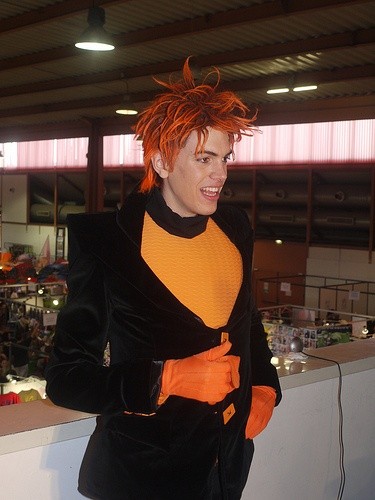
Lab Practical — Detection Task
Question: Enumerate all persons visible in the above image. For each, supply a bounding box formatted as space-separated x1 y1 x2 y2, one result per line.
45 54 283 500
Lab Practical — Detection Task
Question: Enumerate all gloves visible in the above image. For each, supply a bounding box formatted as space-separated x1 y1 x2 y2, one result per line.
246 384 275 439
159 339 242 401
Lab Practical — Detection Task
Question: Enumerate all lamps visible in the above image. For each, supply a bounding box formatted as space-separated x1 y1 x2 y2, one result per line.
73 0 116 54
